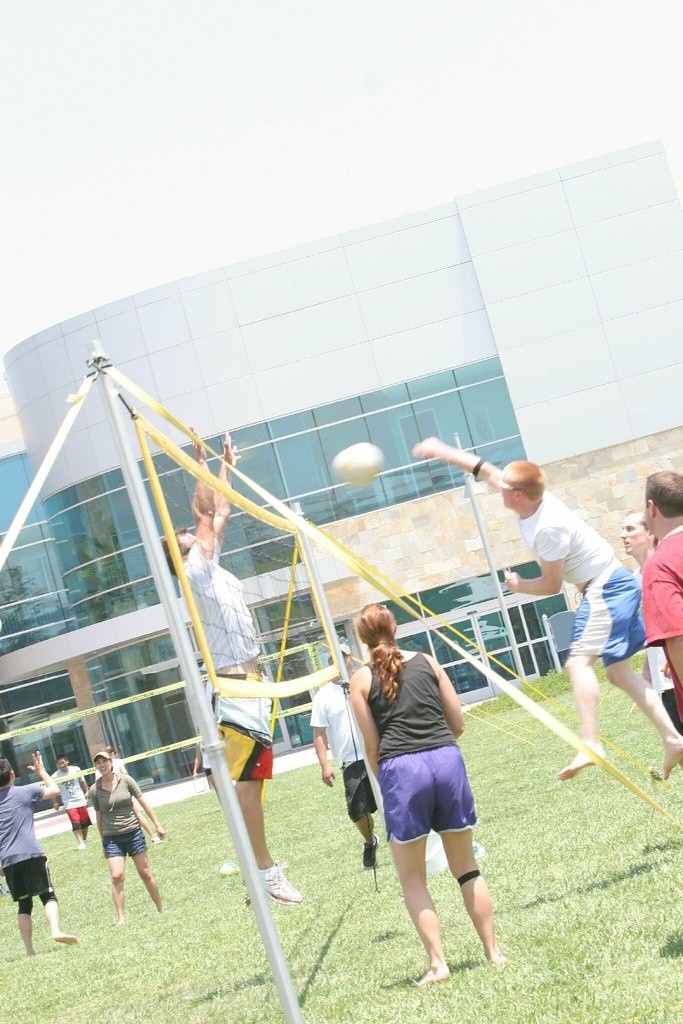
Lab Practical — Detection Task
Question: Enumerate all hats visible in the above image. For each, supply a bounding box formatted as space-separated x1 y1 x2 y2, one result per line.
93 750 111 763
327 643 352 659
0 875 11 896
105 746 117 754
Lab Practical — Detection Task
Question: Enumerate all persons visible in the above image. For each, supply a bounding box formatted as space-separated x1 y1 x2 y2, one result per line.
159 428 304 907
192 736 219 801
85 745 166 924
620 469 683 733
413 436 683 779
50 754 92 849
350 603 505 985
0 750 77 954
310 646 379 867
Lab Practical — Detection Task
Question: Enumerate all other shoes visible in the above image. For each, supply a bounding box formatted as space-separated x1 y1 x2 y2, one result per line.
77 839 87 850
150 835 164 845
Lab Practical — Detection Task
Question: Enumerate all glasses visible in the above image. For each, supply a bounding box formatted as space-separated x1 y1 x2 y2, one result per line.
497 480 524 493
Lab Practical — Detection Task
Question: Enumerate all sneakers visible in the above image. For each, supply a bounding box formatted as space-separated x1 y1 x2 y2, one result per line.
242 860 304 906
363 834 380 870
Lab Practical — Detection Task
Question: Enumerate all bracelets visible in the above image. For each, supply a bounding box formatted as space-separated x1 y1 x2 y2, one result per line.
54 803 58 805
473 459 486 476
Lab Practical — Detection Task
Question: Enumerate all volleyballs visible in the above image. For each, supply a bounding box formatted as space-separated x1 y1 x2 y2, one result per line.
218 860 242 879
329 444 383 494
